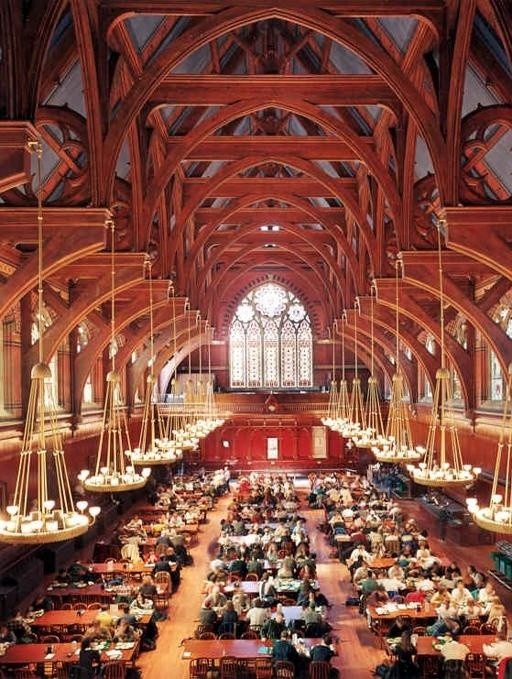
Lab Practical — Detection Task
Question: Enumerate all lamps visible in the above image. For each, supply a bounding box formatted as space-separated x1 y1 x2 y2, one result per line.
0 139 101 545
373 253 427 464
466 362 512 534
77 223 152 494
321 308 384 448
406 219 483 486
124 263 227 464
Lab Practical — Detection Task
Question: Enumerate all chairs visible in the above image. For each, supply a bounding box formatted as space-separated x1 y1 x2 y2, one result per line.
182 473 338 679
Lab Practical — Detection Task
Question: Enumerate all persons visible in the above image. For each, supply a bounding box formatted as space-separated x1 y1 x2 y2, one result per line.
2 462 511 679
